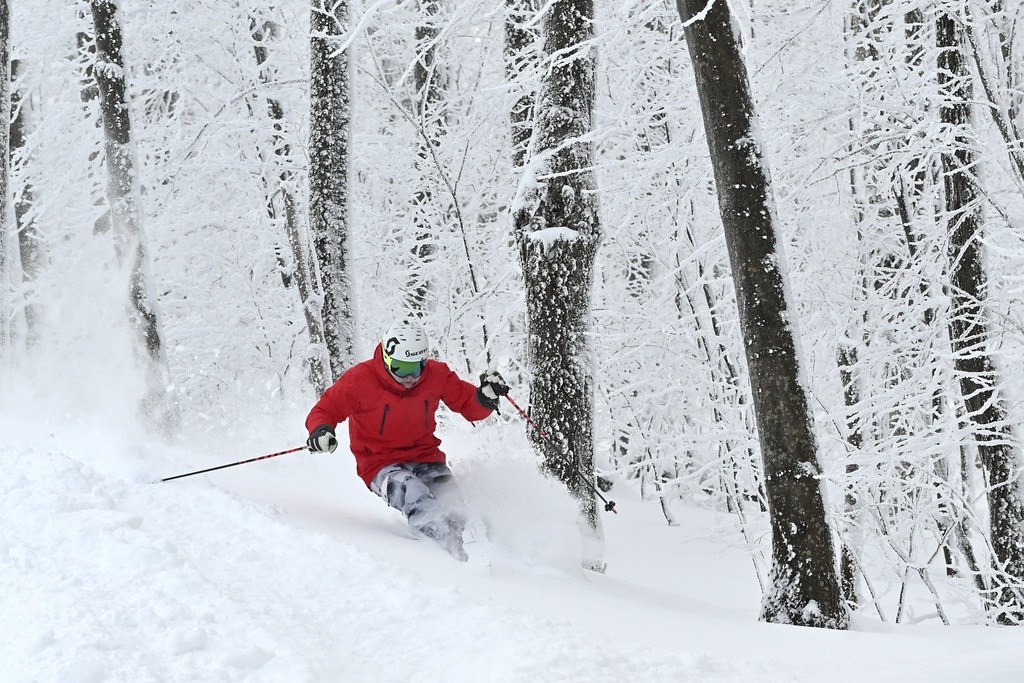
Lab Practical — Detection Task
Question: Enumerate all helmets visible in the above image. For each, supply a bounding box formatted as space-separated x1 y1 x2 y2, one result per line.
382 317 429 362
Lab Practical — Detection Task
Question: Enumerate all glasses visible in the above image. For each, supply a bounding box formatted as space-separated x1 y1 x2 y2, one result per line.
389 358 426 378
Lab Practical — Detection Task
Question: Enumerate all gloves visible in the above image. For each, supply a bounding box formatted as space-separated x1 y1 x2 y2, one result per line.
306 426 338 454
476 369 510 409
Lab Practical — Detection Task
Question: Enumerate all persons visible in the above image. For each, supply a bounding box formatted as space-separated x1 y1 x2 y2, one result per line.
305 321 509 562
